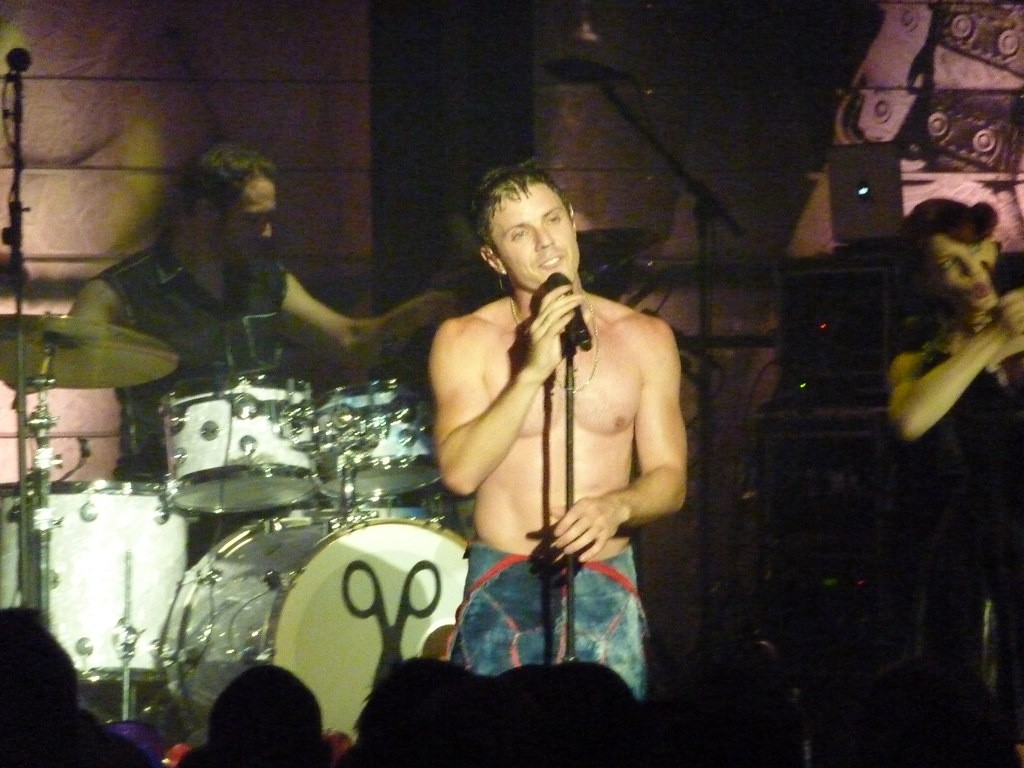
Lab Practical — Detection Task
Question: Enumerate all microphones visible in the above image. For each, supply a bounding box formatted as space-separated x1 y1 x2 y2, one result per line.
543 57 628 84
545 272 592 352
6 47 31 72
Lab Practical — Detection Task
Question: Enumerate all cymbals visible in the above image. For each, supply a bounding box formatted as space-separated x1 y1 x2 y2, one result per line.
0 314 179 389
578 226 660 261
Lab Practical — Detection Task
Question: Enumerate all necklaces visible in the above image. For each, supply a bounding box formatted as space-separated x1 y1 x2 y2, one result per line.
509 289 600 394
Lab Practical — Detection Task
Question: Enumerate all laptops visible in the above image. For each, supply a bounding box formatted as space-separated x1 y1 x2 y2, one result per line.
827 145 908 244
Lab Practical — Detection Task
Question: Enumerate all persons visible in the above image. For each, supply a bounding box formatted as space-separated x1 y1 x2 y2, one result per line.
887 199 1024 672
1 608 1022 766
430 156 689 708
66 142 460 471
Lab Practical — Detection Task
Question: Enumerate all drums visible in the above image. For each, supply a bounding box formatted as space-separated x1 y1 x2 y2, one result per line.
1 479 188 682
159 375 320 514
160 505 468 743
306 380 442 498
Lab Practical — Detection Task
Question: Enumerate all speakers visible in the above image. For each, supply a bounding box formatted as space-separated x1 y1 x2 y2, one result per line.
753 404 942 710
